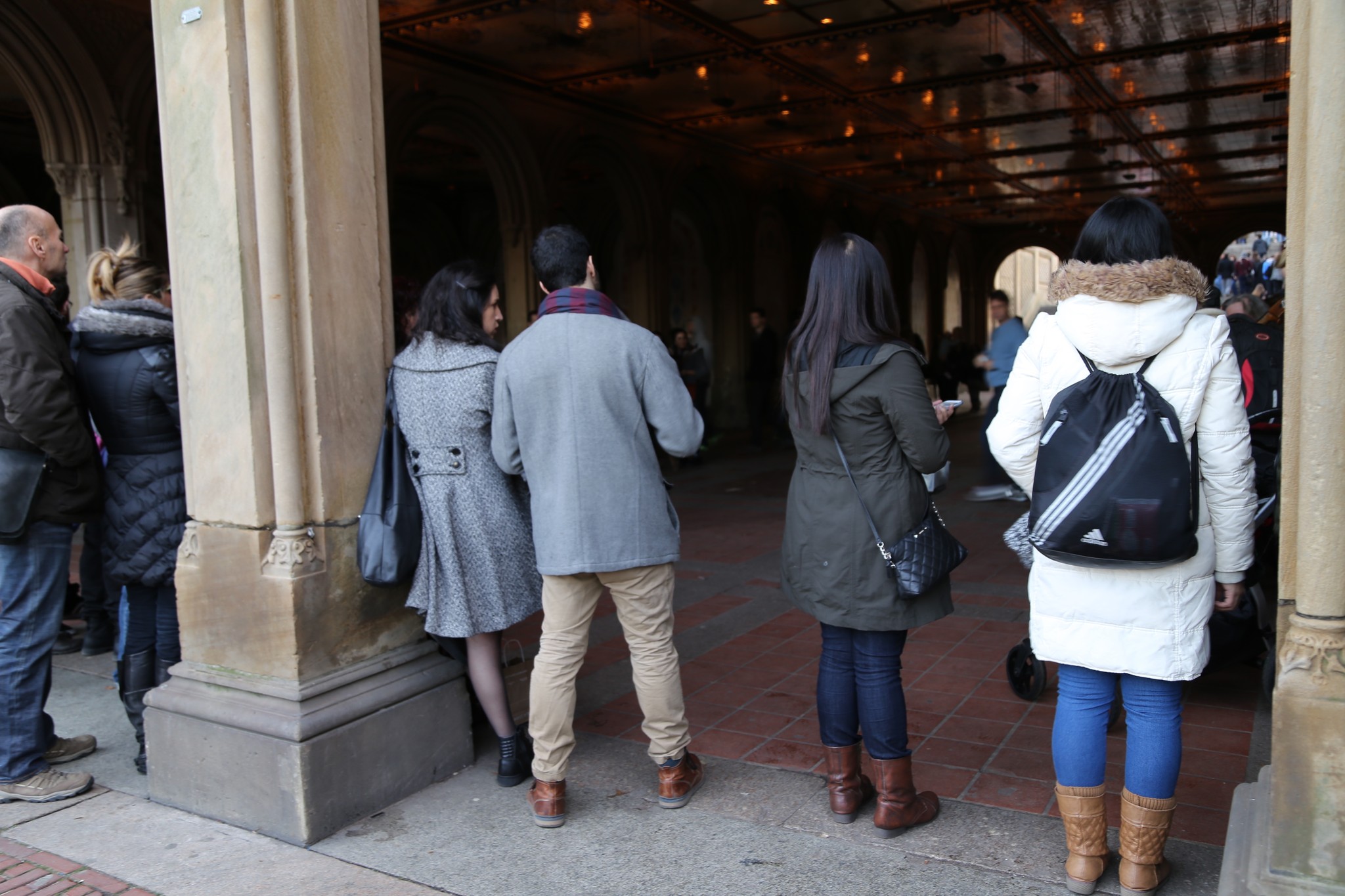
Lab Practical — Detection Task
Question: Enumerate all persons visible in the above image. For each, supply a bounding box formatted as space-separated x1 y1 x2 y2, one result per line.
972 289 1030 502
984 197 1258 896
1204 234 1286 536
394 264 543 787
64 236 186 768
778 234 955 837
0 203 97 801
905 325 990 415
50 278 779 647
491 226 704 830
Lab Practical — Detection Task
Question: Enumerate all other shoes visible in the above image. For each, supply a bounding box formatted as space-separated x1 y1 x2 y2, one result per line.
47 637 82 656
83 634 113 655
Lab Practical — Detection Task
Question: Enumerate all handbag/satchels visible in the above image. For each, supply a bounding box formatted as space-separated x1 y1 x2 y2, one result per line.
886 517 970 598
0 448 53 543
356 410 422 589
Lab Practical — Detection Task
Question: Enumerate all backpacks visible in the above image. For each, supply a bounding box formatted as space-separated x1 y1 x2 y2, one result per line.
1027 352 1201 567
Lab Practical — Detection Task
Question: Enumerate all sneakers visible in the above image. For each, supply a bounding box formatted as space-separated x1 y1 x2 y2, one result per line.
527 777 565 828
657 747 704 810
41 735 96 765
0 769 95 805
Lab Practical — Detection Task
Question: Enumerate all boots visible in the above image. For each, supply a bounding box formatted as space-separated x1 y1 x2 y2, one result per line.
495 728 534 788
871 755 940 839
117 648 175 774
1053 782 1110 895
1117 786 1178 896
821 736 873 825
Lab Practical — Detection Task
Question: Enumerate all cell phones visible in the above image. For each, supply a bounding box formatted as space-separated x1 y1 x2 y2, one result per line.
933 399 963 412
